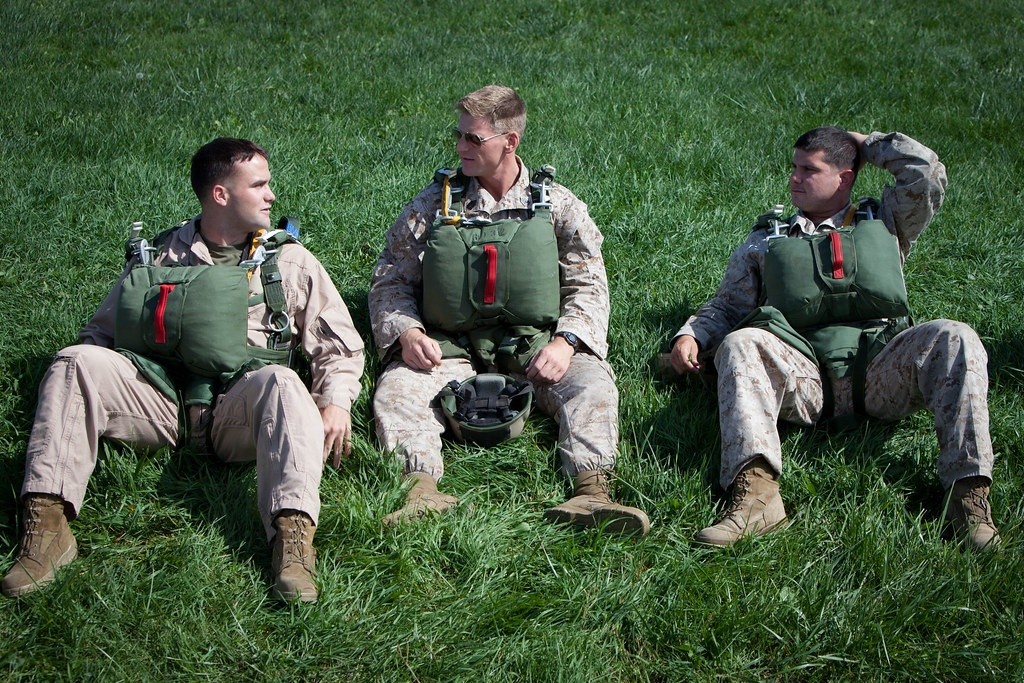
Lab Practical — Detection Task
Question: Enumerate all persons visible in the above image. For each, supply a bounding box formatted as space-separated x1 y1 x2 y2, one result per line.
0 139 364 602
369 83 650 539
671 127 1002 553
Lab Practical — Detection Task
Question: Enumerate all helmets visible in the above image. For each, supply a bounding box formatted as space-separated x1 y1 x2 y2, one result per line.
441 373 533 447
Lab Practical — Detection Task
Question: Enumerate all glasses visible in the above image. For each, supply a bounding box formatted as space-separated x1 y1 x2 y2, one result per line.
451 127 510 148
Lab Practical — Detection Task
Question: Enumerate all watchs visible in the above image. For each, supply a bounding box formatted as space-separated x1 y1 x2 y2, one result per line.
555 332 579 352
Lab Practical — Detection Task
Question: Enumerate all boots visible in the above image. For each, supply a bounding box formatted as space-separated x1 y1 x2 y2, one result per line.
381 471 474 526
941 478 1002 552
1 497 77 597
543 471 650 539
272 516 316 602
698 459 789 545
